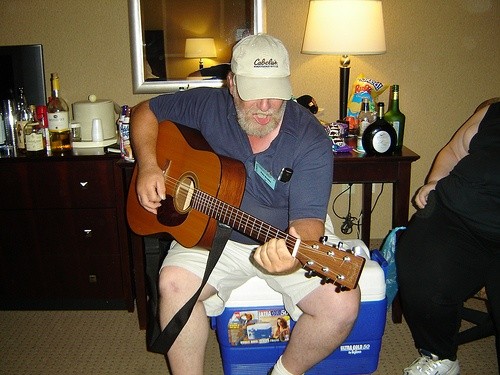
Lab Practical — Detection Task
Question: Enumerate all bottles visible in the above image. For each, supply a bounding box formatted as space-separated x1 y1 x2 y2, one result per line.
72 94 117 141
47 73 73 154
358 98 373 137
70 123 81 142
116 105 131 141
121 141 135 159
362 102 397 157
0 87 51 152
384 85 405 151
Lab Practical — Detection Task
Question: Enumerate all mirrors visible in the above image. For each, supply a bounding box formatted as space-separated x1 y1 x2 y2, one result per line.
128 0 266 94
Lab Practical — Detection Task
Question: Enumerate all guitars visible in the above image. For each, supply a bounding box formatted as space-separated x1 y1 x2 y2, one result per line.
125 120 366 292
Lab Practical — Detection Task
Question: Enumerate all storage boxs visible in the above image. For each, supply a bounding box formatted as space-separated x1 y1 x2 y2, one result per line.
210 238 389 375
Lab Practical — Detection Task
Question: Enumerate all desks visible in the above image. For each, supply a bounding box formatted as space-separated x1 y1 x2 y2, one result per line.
114 145 422 325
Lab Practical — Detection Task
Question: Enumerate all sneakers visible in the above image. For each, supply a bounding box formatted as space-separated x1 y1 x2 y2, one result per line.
402 348 461 375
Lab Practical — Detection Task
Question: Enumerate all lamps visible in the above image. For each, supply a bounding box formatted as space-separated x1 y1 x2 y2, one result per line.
302 0 386 122
183 37 217 69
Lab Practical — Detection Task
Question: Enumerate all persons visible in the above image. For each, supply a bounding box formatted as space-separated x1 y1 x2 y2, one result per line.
394 100 500 375
188 63 231 79
130 33 361 375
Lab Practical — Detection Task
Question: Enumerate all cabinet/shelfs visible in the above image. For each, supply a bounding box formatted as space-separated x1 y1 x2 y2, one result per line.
0 155 136 311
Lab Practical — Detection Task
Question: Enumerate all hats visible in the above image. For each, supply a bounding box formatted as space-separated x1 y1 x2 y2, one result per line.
231 32 292 100
